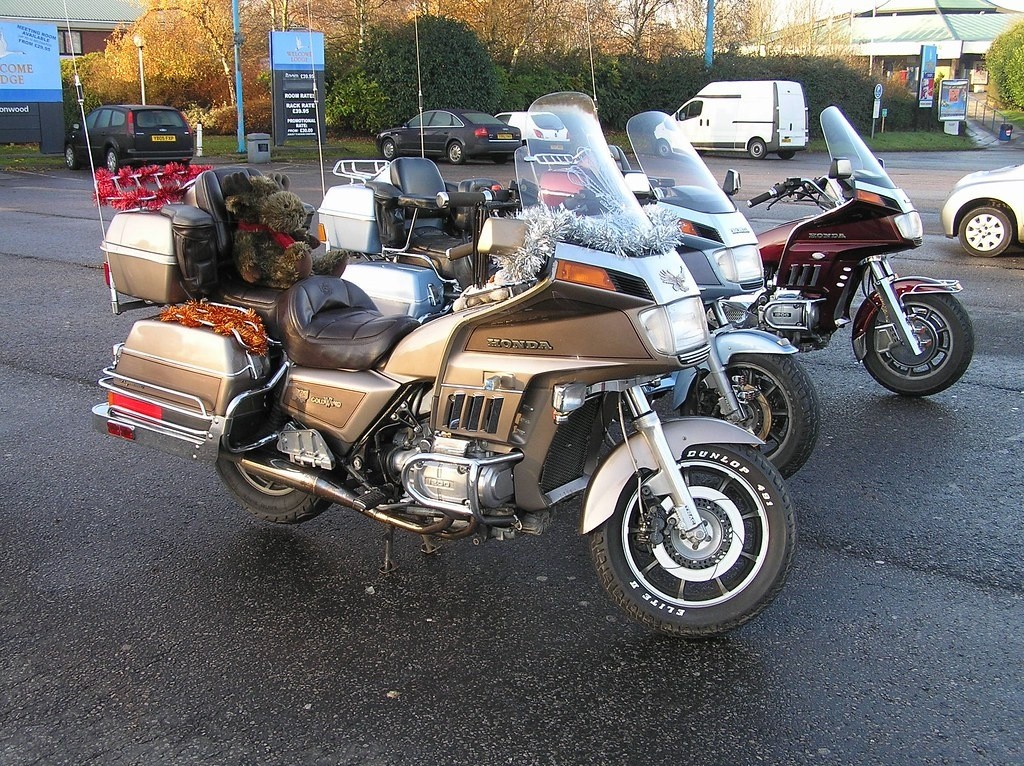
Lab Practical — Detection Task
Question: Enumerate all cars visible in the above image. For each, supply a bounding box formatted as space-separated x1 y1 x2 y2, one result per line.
942 159 1024 257
493 110 571 161
375 109 522 165
64 104 195 177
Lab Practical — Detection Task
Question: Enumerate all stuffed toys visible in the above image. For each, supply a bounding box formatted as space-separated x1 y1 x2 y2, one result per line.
224 174 350 290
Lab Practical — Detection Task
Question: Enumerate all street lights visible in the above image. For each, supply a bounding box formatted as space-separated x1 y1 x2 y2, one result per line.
133 35 147 105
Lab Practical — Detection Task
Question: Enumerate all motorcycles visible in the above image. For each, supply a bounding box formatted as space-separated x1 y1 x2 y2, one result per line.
603 101 975 395
98 88 797 638
318 109 822 486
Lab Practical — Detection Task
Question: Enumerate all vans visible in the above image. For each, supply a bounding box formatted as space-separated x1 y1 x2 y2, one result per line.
652 79 808 161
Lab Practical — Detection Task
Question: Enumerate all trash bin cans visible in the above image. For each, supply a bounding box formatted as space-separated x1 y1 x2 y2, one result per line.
246 133 271 163
998 124 1013 141
